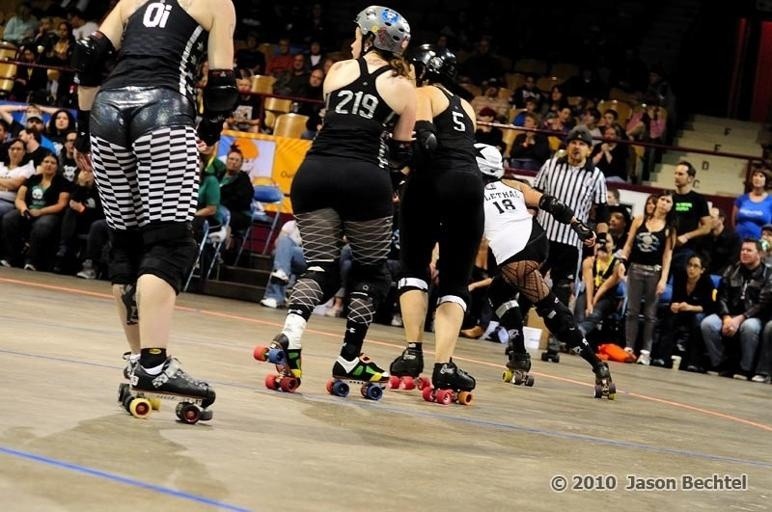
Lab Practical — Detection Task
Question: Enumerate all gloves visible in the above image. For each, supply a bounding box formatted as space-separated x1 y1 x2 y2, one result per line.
571 218 593 243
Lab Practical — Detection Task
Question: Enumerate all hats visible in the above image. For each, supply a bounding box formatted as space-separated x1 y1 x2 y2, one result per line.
26 113 44 120
565 129 591 146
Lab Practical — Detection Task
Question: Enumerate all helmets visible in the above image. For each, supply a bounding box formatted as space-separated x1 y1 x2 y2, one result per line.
357 4 412 57
409 43 461 83
471 142 504 178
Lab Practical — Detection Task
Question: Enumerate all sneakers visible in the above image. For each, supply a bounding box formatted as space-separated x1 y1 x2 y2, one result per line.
1 260 12 267
705 370 727 375
261 297 277 307
325 308 345 315
390 314 404 328
24 263 38 270
638 351 652 364
734 373 752 379
653 360 665 365
688 366 707 372
460 325 484 337
77 260 98 280
752 375 769 382
623 347 633 353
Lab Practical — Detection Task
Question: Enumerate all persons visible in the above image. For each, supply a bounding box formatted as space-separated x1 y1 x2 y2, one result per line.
700 238 772 380
1 153 70 270
752 320 772 382
637 194 658 220
654 252 716 372
598 188 634 224
617 188 676 365
667 160 714 305
758 223 772 268
0 1 675 184
325 236 351 317
732 165 772 242
386 194 401 325
608 206 631 251
169 153 221 295
198 146 255 278
576 230 619 336
468 142 616 401
261 220 304 309
463 261 492 323
505 125 610 362
709 206 741 276
461 295 495 340
54 169 105 279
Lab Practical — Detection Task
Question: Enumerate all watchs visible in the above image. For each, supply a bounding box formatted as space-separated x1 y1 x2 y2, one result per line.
620 259 626 262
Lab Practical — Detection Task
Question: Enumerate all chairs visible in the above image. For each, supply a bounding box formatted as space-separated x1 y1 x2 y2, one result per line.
183 220 211 292
644 284 674 306
264 112 278 126
710 274 723 290
634 106 667 116
535 76 561 91
501 128 525 148
548 136 561 151
498 87 513 100
251 75 279 94
599 101 632 120
460 85 484 97
273 113 310 138
245 184 285 255
264 96 293 113
0 62 17 78
0 48 18 61
0 76 15 92
233 212 254 266
574 281 627 318
201 204 233 281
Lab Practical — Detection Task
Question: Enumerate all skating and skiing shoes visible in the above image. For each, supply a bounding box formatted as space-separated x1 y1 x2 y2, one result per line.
130 356 216 424
255 334 303 391
592 361 617 400
502 352 535 387
388 349 431 392
329 356 389 401
424 360 477 404
542 340 562 364
120 352 161 411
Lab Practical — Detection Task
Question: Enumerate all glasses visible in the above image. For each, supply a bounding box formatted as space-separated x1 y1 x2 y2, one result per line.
685 261 702 271
